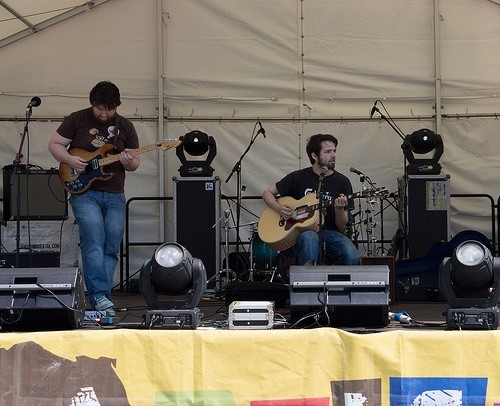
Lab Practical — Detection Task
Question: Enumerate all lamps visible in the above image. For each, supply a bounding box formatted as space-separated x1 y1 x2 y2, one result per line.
175 129 217 176
401 129 447 174
439 239 500 330
140 242 207 329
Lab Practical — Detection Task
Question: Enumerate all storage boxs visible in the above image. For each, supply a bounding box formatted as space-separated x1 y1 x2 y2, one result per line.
228 299 275 330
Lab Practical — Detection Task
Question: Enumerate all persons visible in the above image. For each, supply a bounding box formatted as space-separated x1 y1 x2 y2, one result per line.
262 134 360 265
48 81 140 316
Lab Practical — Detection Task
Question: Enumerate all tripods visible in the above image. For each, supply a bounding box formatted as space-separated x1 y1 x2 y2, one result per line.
203 191 245 287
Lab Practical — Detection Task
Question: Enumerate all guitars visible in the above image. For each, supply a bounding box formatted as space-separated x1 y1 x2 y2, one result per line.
257 186 388 251
59 138 182 194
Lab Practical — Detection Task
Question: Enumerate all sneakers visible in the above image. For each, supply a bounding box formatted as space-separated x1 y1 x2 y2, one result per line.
104 307 116 317
94 294 114 311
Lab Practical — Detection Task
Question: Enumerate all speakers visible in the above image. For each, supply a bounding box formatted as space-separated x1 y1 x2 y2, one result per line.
289 264 391 331
0 267 85 332
2 170 69 220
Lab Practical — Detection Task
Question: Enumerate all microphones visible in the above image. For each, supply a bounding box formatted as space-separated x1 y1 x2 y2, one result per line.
320 165 328 178
350 167 364 176
26 96 42 108
370 101 377 118
259 121 266 139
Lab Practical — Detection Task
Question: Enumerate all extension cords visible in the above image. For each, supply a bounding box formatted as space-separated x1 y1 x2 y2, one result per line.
84 310 107 321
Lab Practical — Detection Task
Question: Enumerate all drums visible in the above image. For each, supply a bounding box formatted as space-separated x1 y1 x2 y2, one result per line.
250 226 279 274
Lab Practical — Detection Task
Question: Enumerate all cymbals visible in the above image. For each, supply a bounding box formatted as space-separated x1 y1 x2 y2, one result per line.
356 195 384 198
